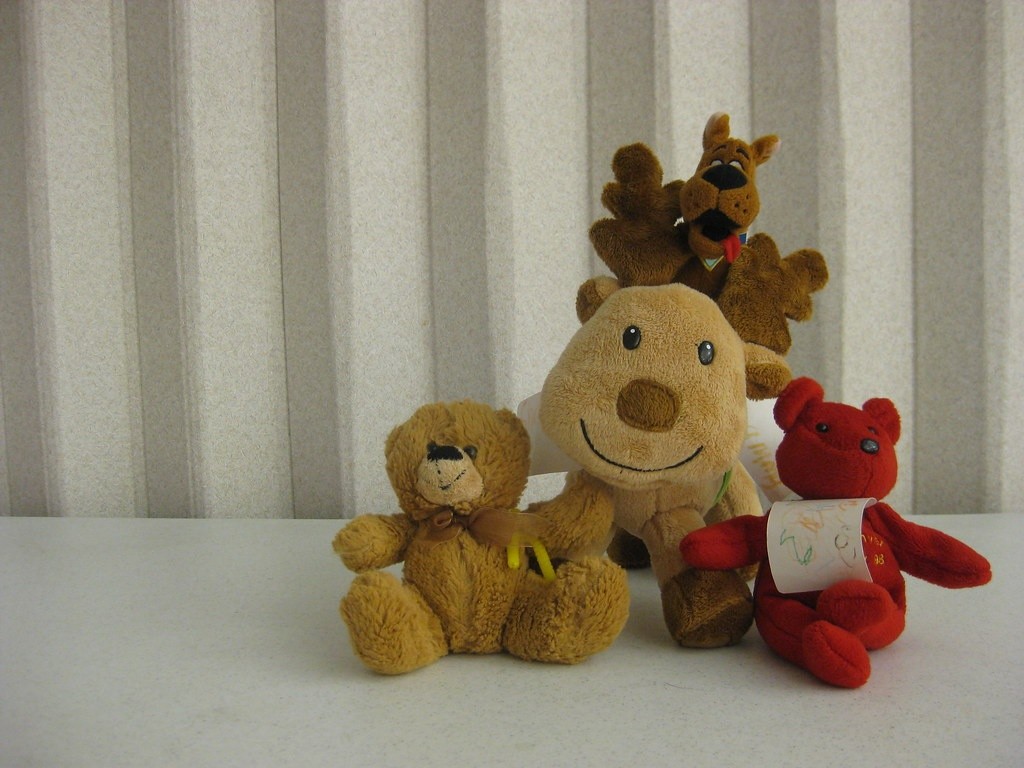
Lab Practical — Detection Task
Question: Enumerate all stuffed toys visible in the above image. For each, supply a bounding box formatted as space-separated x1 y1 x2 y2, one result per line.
327 401 630 674
680 375 993 687
506 113 829 650
